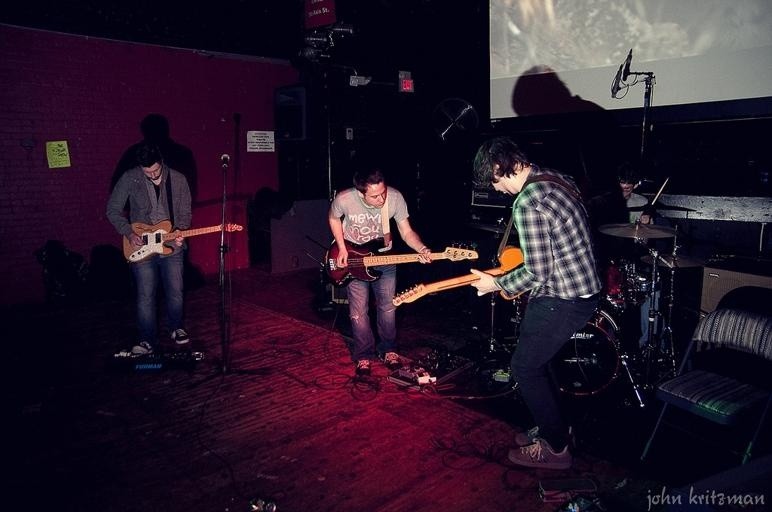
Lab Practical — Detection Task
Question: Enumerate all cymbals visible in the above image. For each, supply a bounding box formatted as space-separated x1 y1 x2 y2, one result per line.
641 250 708 267
621 204 698 213
598 221 676 239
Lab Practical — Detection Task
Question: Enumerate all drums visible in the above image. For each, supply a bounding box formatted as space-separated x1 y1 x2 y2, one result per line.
605 258 629 316
543 309 635 395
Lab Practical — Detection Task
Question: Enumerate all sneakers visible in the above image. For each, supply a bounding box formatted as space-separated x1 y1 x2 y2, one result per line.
132 341 152 354
357 359 370 374
171 329 189 345
508 425 572 469
385 352 402 369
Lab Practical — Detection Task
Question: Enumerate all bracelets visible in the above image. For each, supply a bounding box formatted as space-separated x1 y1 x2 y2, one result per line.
419 246 427 253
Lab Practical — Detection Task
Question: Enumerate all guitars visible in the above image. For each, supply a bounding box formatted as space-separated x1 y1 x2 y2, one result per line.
392 246 525 307
323 238 479 286
123 220 243 261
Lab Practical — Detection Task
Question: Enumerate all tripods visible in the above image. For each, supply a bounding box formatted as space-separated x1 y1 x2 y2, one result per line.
187 198 271 384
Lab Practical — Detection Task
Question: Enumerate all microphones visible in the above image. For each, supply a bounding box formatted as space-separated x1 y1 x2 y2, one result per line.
611 64 621 98
218 151 232 170
623 49 633 81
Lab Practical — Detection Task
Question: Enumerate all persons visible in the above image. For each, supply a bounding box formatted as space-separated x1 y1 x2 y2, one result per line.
105 143 192 355
327 168 433 378
469 136 604 470
587 162 660 350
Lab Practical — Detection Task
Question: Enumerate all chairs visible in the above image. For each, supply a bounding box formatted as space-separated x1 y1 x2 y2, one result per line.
639 309 772 465
